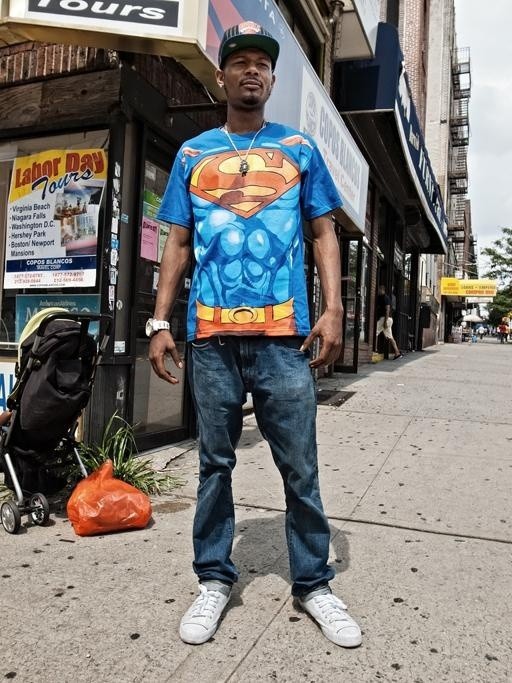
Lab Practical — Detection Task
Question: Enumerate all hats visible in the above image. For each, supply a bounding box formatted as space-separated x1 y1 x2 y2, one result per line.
217 19 282 70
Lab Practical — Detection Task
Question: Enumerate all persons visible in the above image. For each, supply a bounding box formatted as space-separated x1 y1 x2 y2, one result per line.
375 285 403 361
144 21 363 647
478 324 512 345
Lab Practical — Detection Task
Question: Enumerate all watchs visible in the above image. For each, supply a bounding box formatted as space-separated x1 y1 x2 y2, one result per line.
145 318 170 336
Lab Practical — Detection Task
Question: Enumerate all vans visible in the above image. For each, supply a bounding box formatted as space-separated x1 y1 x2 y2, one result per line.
347 295 356 335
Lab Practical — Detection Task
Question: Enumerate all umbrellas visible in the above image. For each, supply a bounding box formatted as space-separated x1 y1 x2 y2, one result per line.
460 314 484 329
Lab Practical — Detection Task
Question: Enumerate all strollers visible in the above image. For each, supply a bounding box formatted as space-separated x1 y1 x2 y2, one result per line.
0 308 117 531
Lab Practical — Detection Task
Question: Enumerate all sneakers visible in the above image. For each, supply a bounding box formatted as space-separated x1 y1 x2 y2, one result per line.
393 354 403 361
177 582 234 648
296 589 364 651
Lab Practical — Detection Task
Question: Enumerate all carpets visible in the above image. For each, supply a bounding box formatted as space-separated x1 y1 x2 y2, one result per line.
313 388 357 407
